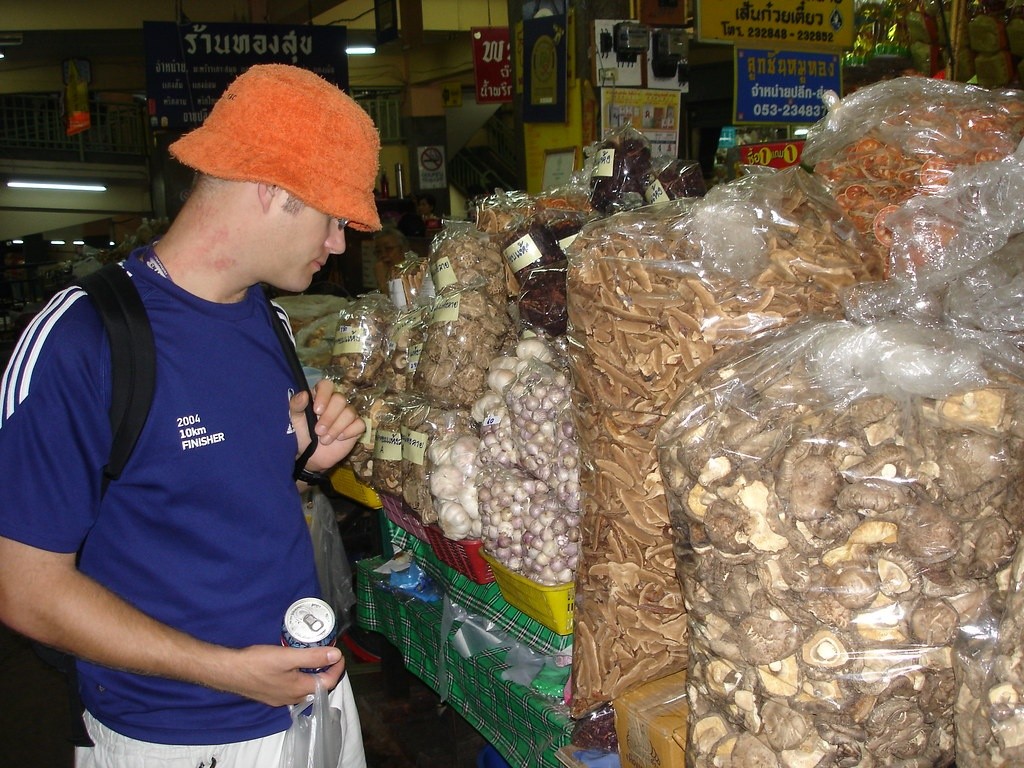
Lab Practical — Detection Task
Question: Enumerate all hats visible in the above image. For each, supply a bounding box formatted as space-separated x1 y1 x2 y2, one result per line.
171 62 383 232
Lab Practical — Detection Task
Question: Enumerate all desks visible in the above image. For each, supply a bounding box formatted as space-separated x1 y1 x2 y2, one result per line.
355 506 581 768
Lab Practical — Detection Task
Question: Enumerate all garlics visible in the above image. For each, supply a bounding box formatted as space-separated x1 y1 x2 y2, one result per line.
426 335 579 586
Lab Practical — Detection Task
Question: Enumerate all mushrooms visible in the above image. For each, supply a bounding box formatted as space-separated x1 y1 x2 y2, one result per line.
657 348 1024 768
304 231 518 525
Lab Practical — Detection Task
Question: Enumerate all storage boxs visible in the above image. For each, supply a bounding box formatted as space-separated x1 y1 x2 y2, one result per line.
611 666 690 768
327 466 577 637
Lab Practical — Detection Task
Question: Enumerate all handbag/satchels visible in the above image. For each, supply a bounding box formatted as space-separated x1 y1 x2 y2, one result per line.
280 676 342 768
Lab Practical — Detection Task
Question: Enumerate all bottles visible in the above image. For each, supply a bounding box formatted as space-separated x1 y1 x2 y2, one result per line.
380 167 389 199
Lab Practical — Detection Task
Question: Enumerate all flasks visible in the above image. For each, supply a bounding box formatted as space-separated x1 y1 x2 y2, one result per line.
395 163 404 199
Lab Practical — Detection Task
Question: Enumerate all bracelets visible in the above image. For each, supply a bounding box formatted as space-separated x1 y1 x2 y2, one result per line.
298 468 334 486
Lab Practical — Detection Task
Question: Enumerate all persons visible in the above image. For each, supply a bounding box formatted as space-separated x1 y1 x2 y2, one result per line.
0 64 381 768
373 227 410 290
397 193 440 237
